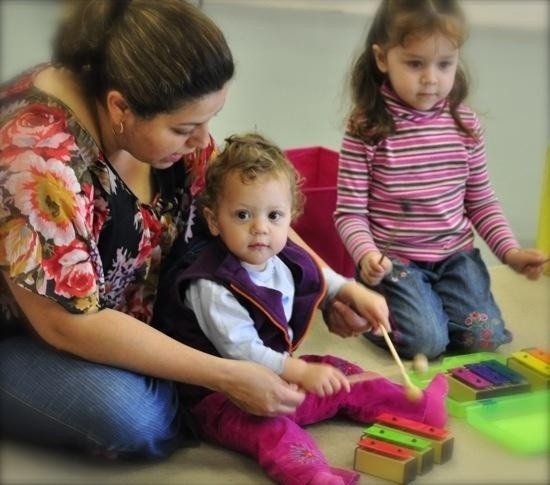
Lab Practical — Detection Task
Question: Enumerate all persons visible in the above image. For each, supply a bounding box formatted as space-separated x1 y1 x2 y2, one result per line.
151 132 449 485
331 0 549 364
1 1 393 469
